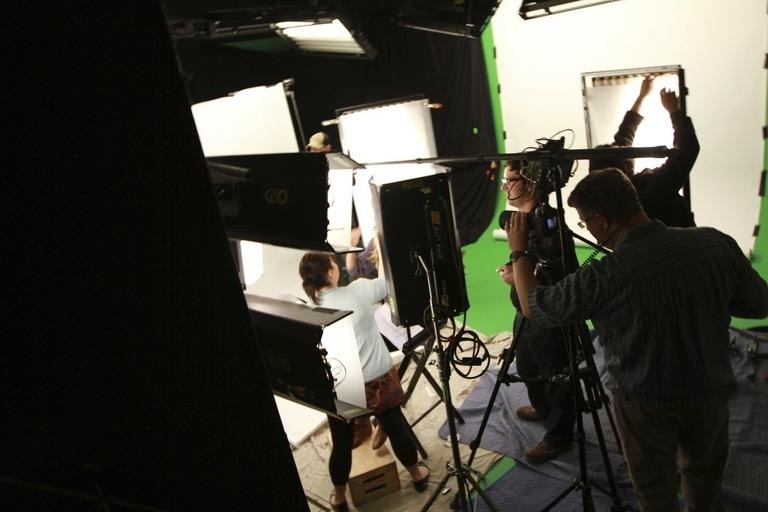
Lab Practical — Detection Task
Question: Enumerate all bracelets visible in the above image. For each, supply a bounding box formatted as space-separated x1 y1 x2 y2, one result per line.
509 251 530 261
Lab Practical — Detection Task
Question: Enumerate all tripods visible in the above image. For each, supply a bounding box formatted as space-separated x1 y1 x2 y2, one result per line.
417 251 498 512
541 166 620 512
449 263 634 512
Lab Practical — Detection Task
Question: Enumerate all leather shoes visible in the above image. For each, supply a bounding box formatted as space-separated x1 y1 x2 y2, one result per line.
412 461 431 492
329 490 348 511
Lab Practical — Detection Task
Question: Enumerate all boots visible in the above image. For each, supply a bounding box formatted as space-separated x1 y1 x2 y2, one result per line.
372 415 388 449
351 417 372 448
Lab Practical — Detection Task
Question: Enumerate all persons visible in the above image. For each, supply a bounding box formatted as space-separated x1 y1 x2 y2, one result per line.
589 76 701 228
299 252 430 512
503 167 768 512
305 132 361 285
496 161 578 465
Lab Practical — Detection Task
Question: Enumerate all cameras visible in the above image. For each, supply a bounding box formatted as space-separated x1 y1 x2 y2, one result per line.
500 206 564 236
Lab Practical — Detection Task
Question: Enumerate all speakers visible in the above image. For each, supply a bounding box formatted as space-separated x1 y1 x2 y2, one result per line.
367 162 470 328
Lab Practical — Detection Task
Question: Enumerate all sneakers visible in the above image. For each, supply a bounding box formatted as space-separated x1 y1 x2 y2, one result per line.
516 404 541 419
525 437 574 464
